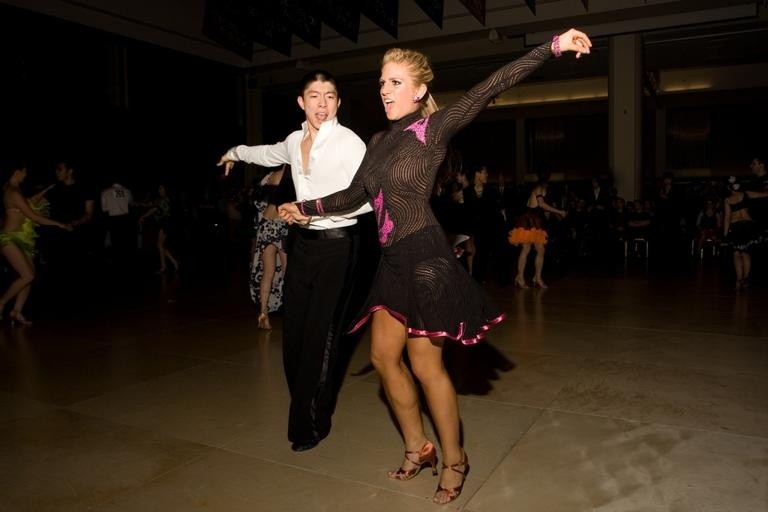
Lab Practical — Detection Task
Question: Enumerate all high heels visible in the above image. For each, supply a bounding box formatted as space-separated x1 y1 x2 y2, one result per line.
514 275 529 289
258 313 272 330
10 309 32 327
388 440 437 481
434 448 467 503
533 275 548 288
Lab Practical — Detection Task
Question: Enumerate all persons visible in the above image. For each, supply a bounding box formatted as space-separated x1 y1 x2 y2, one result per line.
276 26 594 505
452 158 766 290
244 163 297 330
0 158 182 328
216 69 374 452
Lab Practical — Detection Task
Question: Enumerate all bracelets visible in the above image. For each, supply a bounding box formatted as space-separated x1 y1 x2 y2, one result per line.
299 199 309 217
315 197 325 216
551 33 564 60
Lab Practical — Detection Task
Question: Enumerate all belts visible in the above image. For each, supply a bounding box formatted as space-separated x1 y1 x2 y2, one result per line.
303 222 376 240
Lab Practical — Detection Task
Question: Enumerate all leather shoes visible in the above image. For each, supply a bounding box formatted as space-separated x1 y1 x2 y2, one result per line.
292 440 318 451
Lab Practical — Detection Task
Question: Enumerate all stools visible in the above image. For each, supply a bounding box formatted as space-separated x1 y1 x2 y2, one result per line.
620 239 719 264
